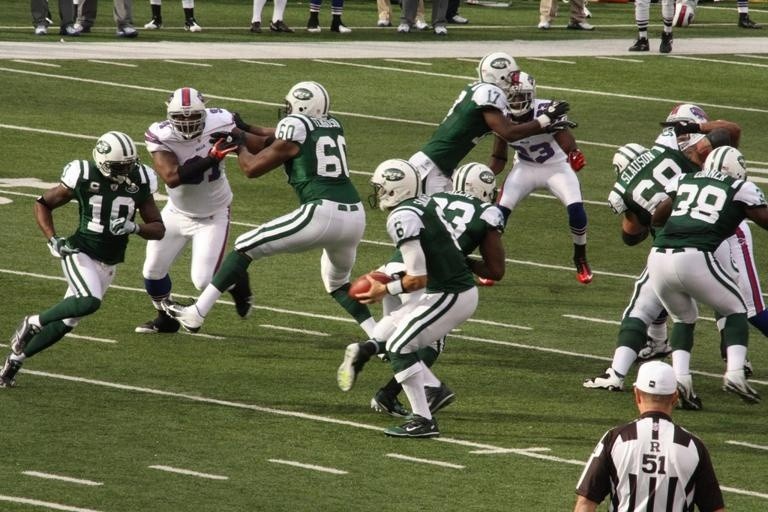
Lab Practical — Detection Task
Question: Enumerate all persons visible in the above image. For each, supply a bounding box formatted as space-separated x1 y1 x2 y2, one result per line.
355 159 480 438
570 360 728 510
252 1 294 32
636 102 768 362
1 132 166 388
628 0 695 54
307 1 351 33
537 0 596 30
645 145 768 411
338 162 506 420
581 142 756 393
737 0 762 28
406 55 578 199
488 71 591 283
29 1 137 37
136 87 276 334
143 1 201 32
159 79 380 337
376 1 468 34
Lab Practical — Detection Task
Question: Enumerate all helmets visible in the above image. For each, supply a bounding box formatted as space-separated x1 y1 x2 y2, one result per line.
611 104 746 179
278 81 331 120
92 131 141 181
165 87 208 141
367 158 423 212
477 54 536 117
452 162 498 205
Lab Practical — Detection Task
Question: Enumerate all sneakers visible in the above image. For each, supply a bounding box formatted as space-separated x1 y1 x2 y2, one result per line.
307 20 321 32
583 6 592 18
336 337 456 437
479 277 494 286
659 38 673 51
33 14 203 37
378 15 471 35
331 23 352 33
270 20 295 33
250 21 262 33
628 37 649 51
738 15 761 29
537 21 550 29
572 256 594 284
584 336 761 411
567 20 595 30
0 273 252 389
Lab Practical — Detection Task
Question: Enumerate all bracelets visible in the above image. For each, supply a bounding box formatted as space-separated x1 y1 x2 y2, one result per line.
387 279 409 297
202 157 215 171
535 113 553 131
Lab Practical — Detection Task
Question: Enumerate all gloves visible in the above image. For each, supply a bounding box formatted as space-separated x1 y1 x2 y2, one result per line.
47 234 79 258
231 112 250 131
205 131 243 164
109 216 141 236
567 147 586 171
542 99 571 124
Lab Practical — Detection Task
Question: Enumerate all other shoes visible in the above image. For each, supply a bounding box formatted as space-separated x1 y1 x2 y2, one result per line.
544 120 578 133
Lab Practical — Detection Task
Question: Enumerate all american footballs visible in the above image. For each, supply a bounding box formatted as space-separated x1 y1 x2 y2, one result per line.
348 271 393 301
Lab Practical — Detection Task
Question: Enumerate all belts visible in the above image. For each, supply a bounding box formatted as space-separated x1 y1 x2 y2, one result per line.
307 198 359 212
